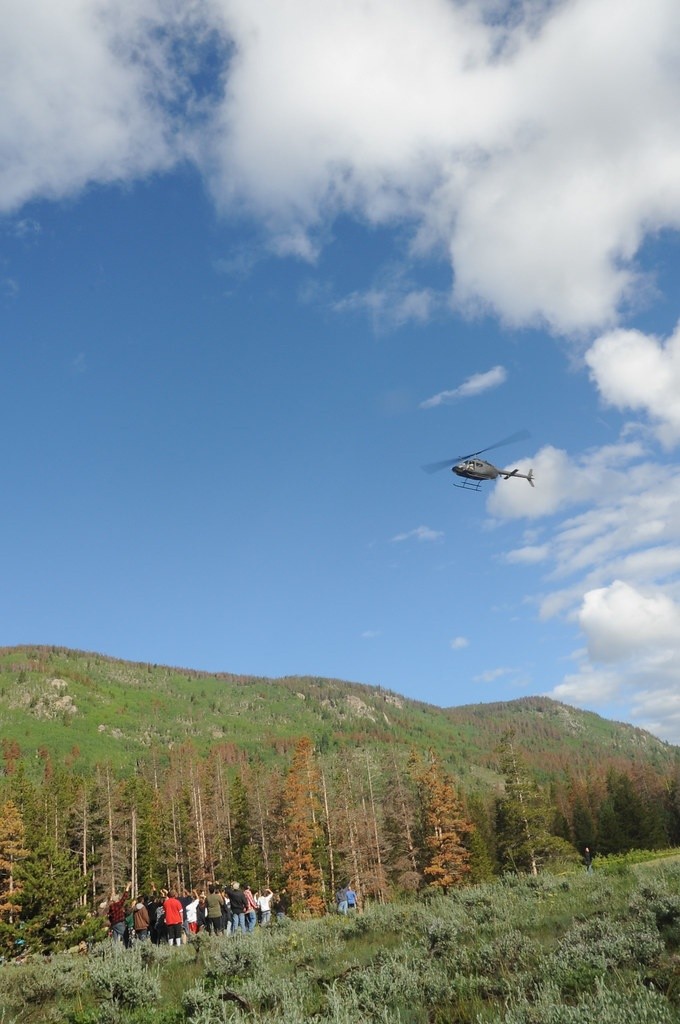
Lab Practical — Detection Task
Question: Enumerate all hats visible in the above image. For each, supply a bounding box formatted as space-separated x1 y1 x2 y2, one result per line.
233 882 240 889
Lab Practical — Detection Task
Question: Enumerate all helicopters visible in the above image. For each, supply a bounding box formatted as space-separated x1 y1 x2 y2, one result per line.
420 429 535 493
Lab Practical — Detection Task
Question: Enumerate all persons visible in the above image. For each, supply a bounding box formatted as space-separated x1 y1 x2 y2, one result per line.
131 896 149 941
109 882 131 951
206 884 223 936
147 896 163 944
335 879 353 915
273 895 286 922
163 890 183 946
226 881 247 935
185 889 199 935
258 889 274 925
123 891 233 939
244 886 258 931
346 887 357 909
